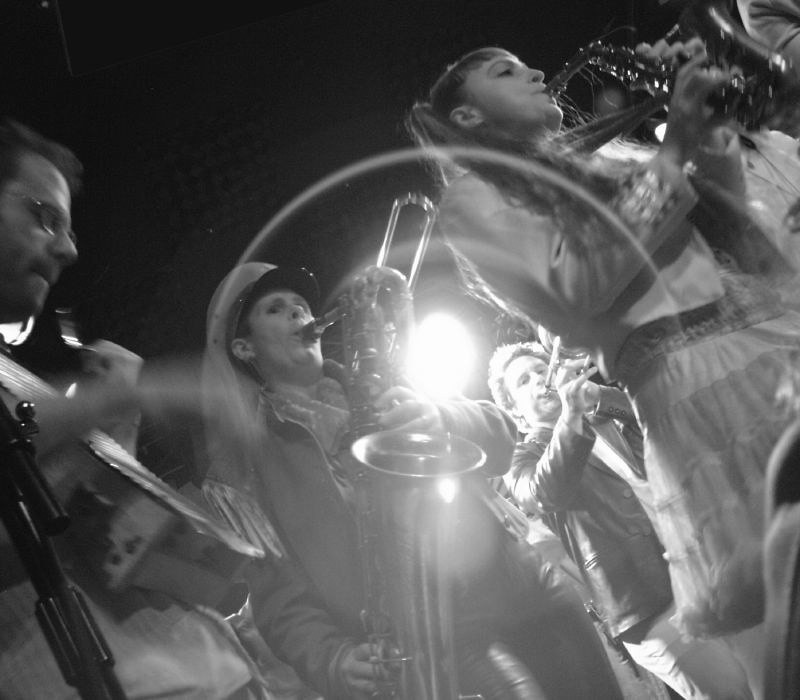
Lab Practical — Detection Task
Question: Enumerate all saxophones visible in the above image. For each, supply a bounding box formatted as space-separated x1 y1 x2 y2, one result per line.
541 0 794 121
290 296 492 700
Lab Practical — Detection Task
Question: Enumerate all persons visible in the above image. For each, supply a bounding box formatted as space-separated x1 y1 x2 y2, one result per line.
199 262 623 700
406 36 800 700
0 116 256 700
486 341 755 700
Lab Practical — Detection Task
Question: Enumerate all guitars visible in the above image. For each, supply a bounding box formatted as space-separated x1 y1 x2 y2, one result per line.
0 349 288 616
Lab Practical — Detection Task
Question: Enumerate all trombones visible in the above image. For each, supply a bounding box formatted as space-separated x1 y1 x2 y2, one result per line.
346 187 440 382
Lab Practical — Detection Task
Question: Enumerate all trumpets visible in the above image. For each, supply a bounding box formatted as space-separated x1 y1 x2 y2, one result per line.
535 320 593 401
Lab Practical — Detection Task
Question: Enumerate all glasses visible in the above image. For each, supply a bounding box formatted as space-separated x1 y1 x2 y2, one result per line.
0 188 78 247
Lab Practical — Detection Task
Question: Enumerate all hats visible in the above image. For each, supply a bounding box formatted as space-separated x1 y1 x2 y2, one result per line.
227 267 321 373
